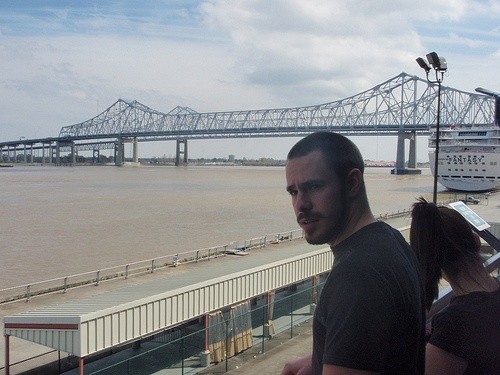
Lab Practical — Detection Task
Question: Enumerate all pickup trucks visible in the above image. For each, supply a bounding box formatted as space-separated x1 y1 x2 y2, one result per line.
459 196 479 205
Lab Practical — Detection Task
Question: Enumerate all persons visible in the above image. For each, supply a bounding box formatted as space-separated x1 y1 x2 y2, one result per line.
410 197 500 375
282 132 426 375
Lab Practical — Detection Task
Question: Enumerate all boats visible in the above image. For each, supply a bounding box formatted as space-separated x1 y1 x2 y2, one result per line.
429 126 500 191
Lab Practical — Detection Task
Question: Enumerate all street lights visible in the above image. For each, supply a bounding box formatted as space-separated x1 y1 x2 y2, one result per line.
416 53 448 206
